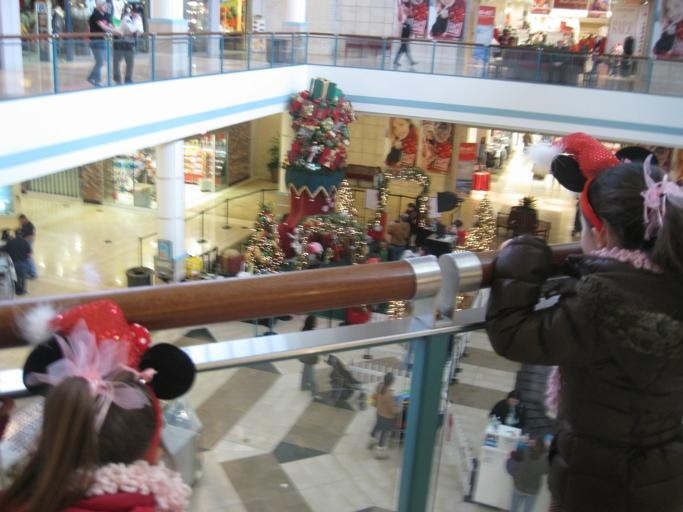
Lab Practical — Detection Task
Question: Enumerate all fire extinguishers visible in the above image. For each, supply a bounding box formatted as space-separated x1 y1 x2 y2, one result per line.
473 166 490 190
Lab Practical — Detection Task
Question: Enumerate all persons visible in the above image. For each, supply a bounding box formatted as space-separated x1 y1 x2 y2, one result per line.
610 41 624 76
391 14 419 69
481 161 681 511
588 36 607 73
16 212 37 282
110 6 147 84
84 0 125 89
0 366 196 511
496 28 513 57
487 386 531 434
271 187 478 461
507 429 550 511
0 228 32 296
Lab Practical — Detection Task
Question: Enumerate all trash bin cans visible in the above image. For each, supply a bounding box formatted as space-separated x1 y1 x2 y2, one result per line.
125 266 155 287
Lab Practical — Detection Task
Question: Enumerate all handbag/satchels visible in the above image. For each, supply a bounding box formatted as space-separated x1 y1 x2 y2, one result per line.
369 393 379 407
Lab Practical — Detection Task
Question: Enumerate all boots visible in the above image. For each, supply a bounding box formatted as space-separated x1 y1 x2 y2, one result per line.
368 438 387 458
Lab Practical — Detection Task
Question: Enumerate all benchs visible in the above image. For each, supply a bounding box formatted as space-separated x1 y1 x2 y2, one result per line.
496 213 551 241
